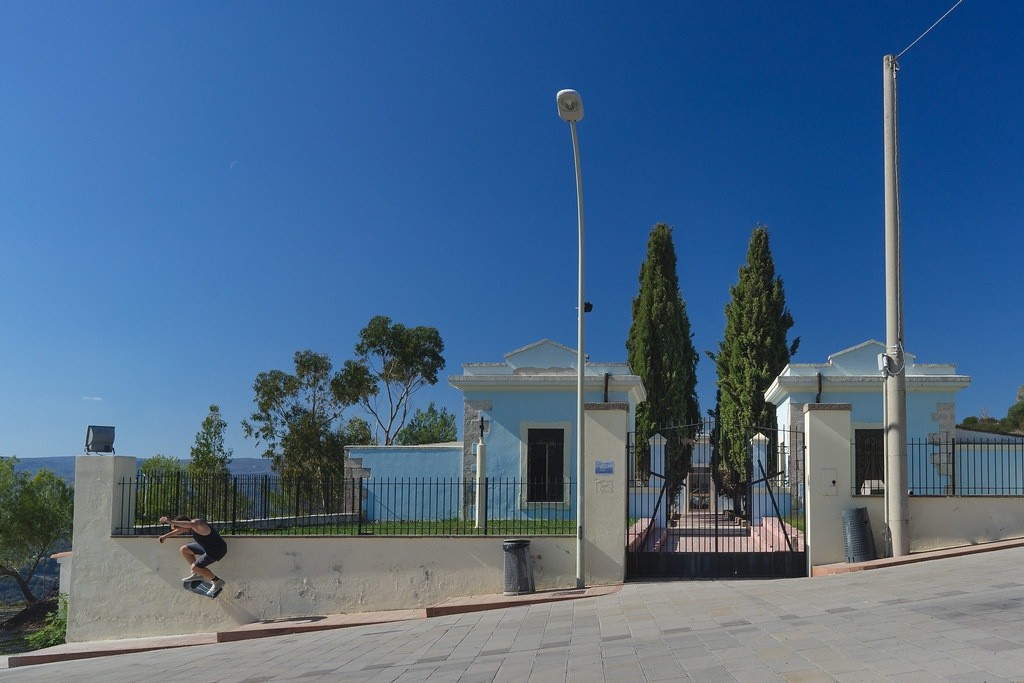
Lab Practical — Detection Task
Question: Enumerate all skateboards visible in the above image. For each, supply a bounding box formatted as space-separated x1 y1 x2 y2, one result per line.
183 579 223 600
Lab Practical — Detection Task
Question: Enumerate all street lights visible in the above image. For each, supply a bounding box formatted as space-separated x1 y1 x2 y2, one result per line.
557 88 587 588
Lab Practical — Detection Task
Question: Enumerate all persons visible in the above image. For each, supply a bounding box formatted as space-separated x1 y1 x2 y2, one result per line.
158 515 227 596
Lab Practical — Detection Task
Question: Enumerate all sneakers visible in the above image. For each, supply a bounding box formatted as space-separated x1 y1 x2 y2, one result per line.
206 577 225 595
183 572 203 582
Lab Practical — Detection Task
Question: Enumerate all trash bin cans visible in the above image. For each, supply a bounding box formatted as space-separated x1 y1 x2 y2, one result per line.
842 507 876 563
502 539 535 595
